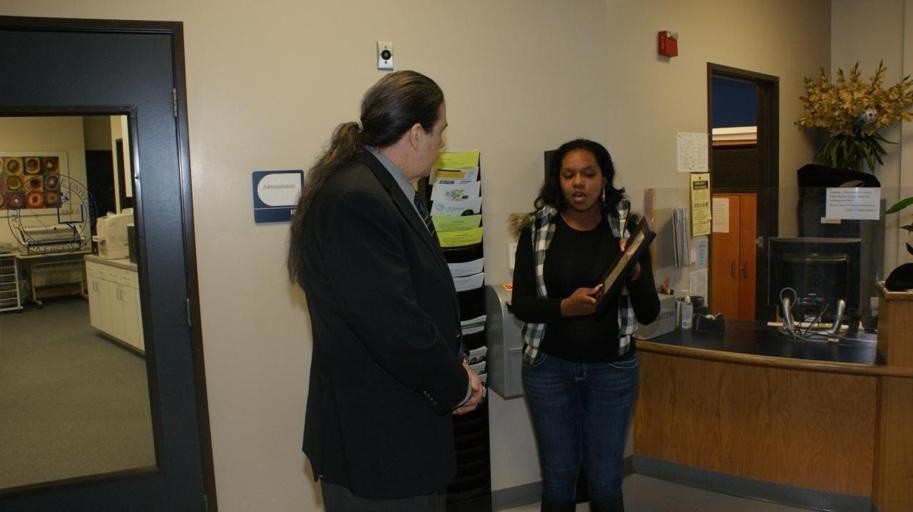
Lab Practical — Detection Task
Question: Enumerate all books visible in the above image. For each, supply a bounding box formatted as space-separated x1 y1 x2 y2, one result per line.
710 126 755 146
423 150 489 385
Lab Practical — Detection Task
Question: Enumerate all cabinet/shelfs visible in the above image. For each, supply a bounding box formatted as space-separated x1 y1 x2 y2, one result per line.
0 254 24 313
112 267 145 352
709 193 758 323
85 258 113 337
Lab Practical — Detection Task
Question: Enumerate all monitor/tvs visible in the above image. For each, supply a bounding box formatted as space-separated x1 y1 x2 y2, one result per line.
767 236 862 343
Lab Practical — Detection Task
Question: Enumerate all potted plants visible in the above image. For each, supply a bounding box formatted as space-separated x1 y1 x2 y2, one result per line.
793 60 913 174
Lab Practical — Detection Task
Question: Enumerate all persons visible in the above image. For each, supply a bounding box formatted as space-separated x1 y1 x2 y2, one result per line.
512 140 661 512
288 71 483 512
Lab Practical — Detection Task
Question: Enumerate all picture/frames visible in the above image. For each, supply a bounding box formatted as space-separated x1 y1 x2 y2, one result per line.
0 149 71 219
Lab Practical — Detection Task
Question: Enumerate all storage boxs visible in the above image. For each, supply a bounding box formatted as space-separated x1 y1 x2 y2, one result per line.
636 293 677 341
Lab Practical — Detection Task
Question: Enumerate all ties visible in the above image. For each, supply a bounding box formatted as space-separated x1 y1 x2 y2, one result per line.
413 192 435 236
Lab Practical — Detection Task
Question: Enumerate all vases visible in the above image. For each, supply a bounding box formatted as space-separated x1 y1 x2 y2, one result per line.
837 132 859 171
